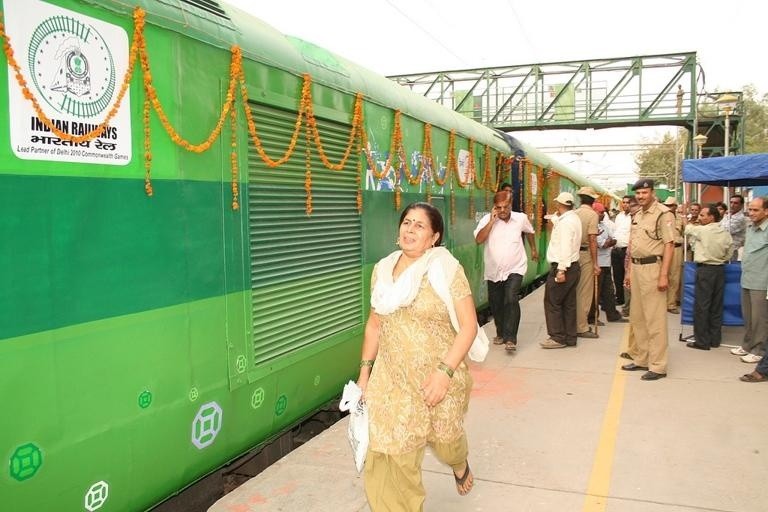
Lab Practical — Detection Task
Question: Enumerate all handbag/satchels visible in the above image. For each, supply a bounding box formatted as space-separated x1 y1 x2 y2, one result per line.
338 380 369 473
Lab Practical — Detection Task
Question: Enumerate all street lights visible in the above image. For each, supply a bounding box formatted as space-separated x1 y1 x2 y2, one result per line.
714 94 740 204
692 133 708 205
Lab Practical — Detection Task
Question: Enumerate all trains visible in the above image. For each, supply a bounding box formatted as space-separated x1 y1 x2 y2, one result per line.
0 0 627 512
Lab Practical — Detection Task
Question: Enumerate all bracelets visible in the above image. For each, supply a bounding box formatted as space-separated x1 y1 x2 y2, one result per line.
359 360 374 369
437 361 454 379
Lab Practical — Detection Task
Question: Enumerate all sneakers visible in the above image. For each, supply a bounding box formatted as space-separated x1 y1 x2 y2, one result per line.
730 346 749 356
708 341 720 347
540 336 568 349
739 353 763 363
687 342 710 350
494 336 504 344
505 340 517 350
577 331 598 339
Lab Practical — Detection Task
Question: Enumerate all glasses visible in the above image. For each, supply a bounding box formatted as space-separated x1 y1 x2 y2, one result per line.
623 202 630 204
629 204 638 209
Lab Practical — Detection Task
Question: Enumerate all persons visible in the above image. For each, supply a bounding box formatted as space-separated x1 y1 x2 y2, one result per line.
473 190 539 354
500 182 512 194
540 179 768 382
351 201 491 512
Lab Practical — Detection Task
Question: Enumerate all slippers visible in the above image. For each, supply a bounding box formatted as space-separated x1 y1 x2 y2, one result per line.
739 373 768 382
453 459 474 496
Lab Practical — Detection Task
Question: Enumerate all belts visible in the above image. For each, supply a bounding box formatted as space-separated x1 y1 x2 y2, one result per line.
674 243 681 247
552 263 557 265
580 247 589 251
617 247 626 251
697 263 724 267
631 256 662 264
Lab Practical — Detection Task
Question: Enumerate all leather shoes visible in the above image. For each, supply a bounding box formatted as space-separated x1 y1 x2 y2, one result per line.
615 300 619 305
593 320 604 326
641 370 667 380
668 309 680 314
621 352 633 360
608 318 629 323
621 363 649 371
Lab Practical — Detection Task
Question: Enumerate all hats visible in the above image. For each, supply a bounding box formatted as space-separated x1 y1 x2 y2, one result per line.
576 186 601 199
553 192 574 206
591 203 606 212
631 178 653 191
663 195 678 206
